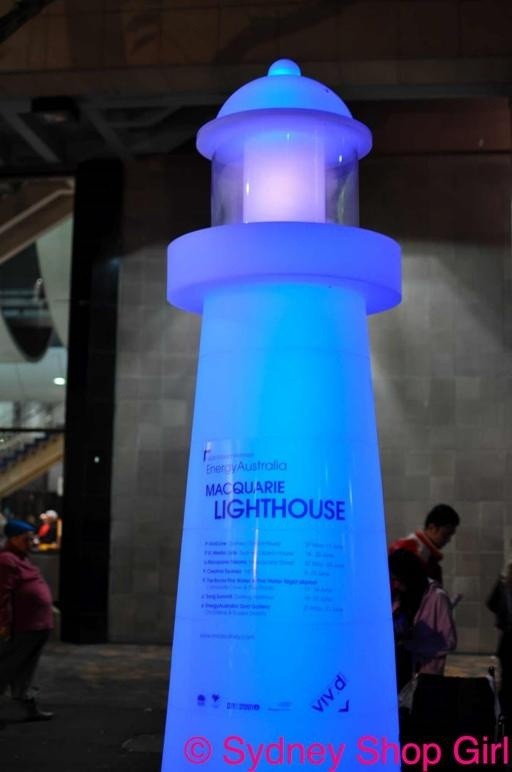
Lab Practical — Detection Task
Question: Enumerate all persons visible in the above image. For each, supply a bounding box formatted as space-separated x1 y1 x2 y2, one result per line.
33 509 58 543
388 547 457 714
484 562 511 737
0 520 55 720
389 504 460 587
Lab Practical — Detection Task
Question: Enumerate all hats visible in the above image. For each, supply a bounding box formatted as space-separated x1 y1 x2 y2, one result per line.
4 519 32 537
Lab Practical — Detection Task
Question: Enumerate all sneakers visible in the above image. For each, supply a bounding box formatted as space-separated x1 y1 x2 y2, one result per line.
20 709 55 721
